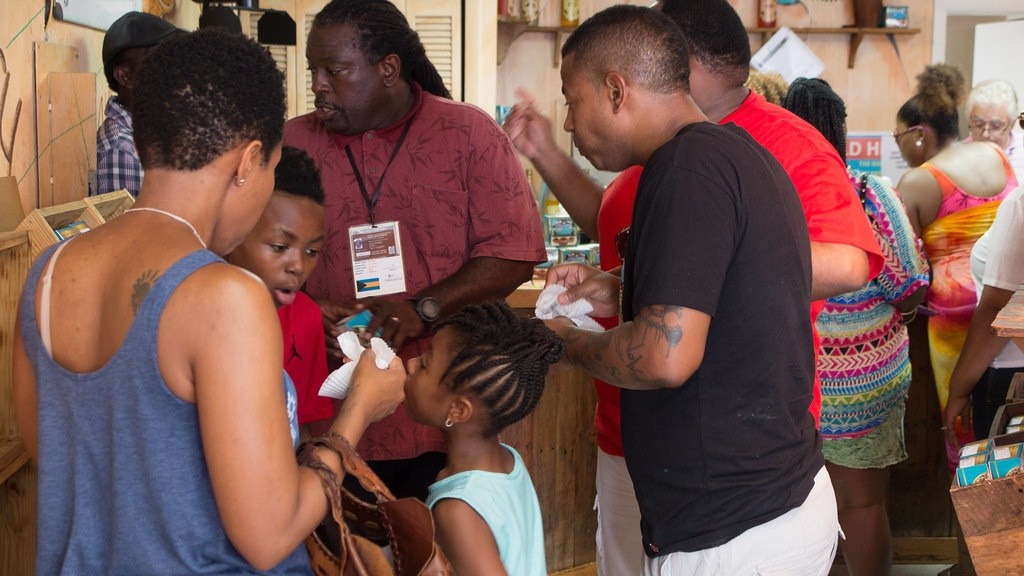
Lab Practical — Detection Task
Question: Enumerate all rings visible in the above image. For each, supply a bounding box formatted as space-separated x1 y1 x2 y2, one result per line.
390 316 398 321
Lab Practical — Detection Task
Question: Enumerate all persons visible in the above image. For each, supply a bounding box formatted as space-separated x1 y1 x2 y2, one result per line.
13 28 407 576
405 300 561 576
225 144 334 442
502 0 886 576
98 11 173 200
545 5 845 576
283 0 548 503
751 64 1024 576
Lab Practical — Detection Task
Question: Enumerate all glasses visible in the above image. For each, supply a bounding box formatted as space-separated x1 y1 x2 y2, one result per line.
893 124 927 142
974 119 1019 135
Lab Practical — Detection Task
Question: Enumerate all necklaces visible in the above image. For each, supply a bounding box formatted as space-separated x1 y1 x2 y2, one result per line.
122 207 207 249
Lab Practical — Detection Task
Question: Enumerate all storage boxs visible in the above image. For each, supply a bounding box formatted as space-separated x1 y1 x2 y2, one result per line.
949 370 1024 576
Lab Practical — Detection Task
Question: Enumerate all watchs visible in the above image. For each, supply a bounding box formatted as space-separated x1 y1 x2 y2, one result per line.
408 295 443 331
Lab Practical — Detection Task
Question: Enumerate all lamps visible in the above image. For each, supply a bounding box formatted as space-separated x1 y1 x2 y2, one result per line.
192 0 297 44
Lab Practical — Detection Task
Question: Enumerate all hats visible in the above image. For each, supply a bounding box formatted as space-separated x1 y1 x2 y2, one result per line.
102 10 189 56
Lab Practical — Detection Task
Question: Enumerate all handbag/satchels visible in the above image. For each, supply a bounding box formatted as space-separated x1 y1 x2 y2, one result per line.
295 433 457 576
